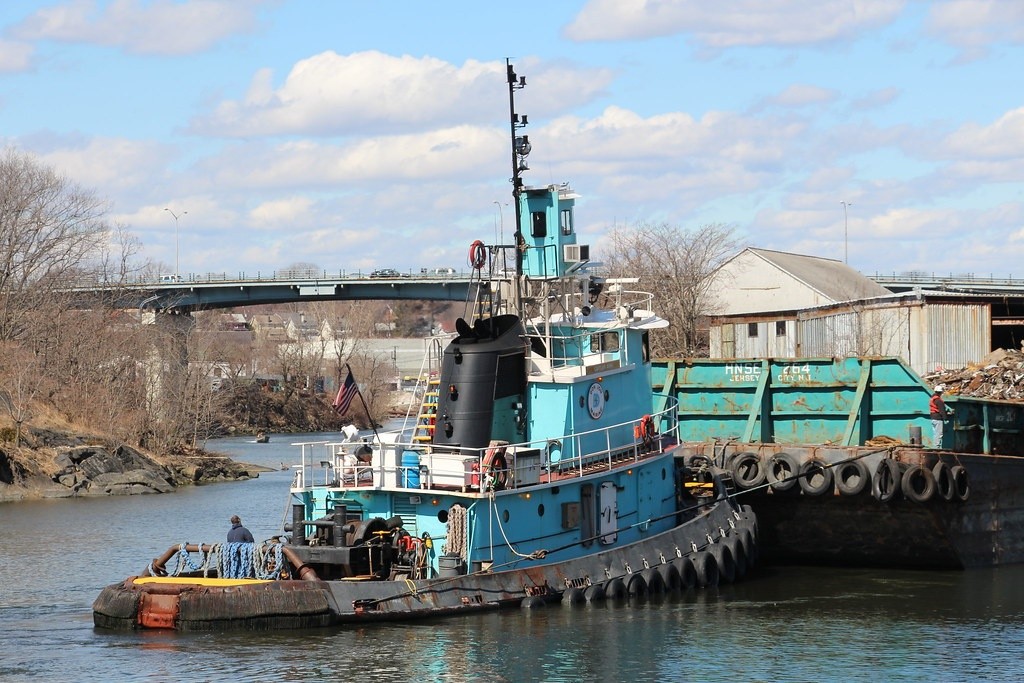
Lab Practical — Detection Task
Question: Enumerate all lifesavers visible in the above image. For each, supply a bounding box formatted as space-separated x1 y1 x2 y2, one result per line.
691 551 720 586
706 504 761 583
470 240 487 270
950 465 971 503
765 453 798 491
835 459 870 496
901 465 936 504
672 558 697 588
640 414 655 441
520 597 548 609
560 588 586 607
932 461 954 503
870 457 915 503
622 573 649 596
799 458 833 497
659 564 681 592
585 584 605 602
641 568 665 594
733 451 766 489
603 578 627 600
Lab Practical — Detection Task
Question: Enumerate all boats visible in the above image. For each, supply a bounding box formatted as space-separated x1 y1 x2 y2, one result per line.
647 352 1024 571
292 58 681 578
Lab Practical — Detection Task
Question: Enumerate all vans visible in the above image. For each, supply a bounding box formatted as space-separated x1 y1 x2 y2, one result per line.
160 275 184 283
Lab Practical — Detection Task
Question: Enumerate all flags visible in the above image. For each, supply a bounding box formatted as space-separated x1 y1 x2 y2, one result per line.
332 371 359 418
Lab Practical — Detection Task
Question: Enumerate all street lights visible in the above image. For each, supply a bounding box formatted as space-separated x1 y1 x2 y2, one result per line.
164 207 187 282
493 200 509 244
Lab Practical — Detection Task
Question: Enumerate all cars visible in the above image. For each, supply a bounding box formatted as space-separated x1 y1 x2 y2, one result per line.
370 269 410 278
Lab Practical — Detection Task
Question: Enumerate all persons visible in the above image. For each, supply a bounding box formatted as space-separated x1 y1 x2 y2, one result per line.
930 386 948 449
227 516 255 543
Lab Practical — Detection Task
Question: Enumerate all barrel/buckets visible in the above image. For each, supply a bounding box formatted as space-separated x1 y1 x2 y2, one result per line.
402 449 426 488
439 556 463 577
